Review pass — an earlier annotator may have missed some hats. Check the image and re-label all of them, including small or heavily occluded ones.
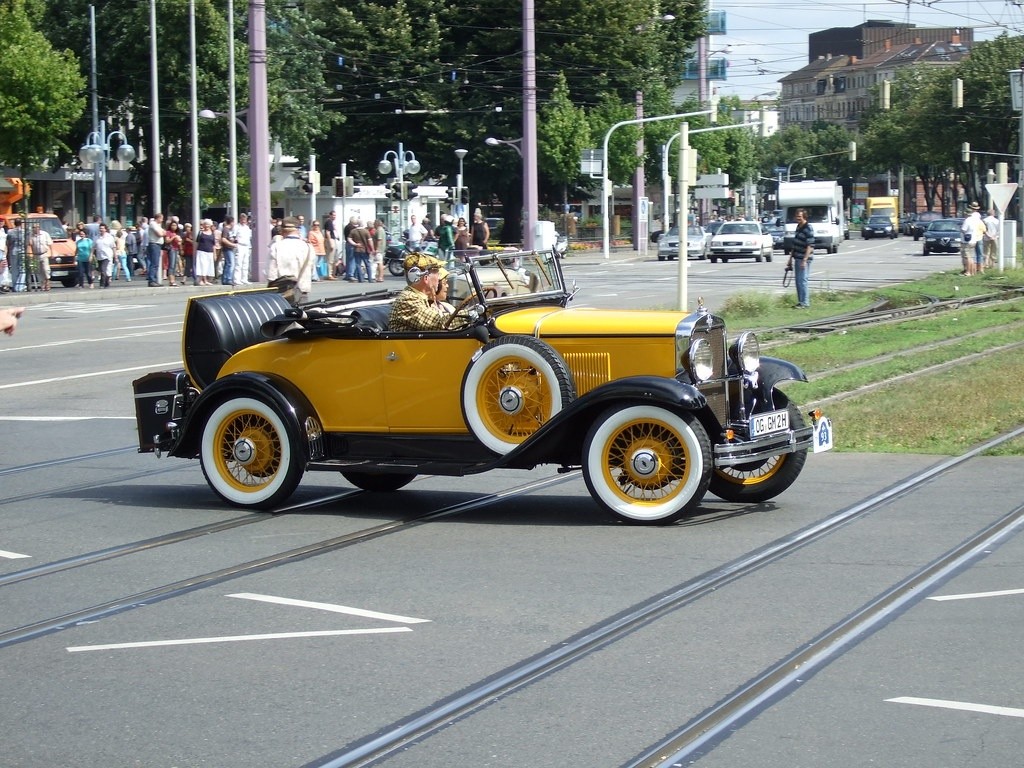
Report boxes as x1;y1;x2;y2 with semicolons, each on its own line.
969;201;981;209
964;208;974;215
444;215;455;223
402;251;448;270
281;216;302;229
110;220;122;229
438;267;450;280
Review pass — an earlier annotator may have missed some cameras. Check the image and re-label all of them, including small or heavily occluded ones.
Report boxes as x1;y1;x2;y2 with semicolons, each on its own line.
785;266;793;272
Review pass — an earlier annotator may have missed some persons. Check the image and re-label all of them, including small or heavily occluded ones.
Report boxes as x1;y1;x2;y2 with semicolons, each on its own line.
905;210;922;222
388;254;485;331
688;196;774;235
656;215;662;222
958;202;999;277
408;207;498;271
267;210;386;283
0;210;254;295
506;257;532;295
787;208;815;310
0;307;26;336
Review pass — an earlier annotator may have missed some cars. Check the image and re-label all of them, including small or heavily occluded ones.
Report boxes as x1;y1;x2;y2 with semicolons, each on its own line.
132;245;833;527
656;210;785;264
844;211;967;255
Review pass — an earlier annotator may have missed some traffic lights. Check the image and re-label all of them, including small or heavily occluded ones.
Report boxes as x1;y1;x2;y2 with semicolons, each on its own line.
403;181;418;201
445;187;455;205
297;171;320;194
392;182;404;200
460;187;469;205
346;177;363;196
385;183;396;201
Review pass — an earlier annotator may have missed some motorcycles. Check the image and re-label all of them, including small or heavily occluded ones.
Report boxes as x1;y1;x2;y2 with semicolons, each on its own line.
383;229;440;276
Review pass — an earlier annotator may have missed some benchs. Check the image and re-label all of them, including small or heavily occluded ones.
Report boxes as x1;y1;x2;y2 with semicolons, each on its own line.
351;303;394;332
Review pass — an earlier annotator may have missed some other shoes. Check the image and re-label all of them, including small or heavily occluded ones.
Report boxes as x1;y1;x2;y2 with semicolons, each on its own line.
0;287;10;293
42;287;51;291
169;282;177;286
976;269;985;275
181;281;185;285
199;281;213;285
139;269;147;274
222;280;236;285
148;281;160;287
959;271;970;276
347;276;358;281
792;302;809;308
233;280;243;284
241;280;253;284
328;274;338;280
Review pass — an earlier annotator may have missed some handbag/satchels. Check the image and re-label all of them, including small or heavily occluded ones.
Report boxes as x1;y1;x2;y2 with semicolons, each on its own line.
964;233;972;241
161;241;172;251
268;274;302;306
365;244;371;254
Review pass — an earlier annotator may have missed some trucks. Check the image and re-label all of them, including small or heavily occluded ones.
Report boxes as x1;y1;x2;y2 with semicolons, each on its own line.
0;177;80;288
863;197;899;238
778;179;845;254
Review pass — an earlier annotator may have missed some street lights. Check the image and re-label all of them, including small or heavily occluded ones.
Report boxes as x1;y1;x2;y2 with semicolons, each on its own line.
485;138;539;251
455;149;468;188
700;47;734;103
79;120;136;225
198;109;271;284
379;143;420;237
